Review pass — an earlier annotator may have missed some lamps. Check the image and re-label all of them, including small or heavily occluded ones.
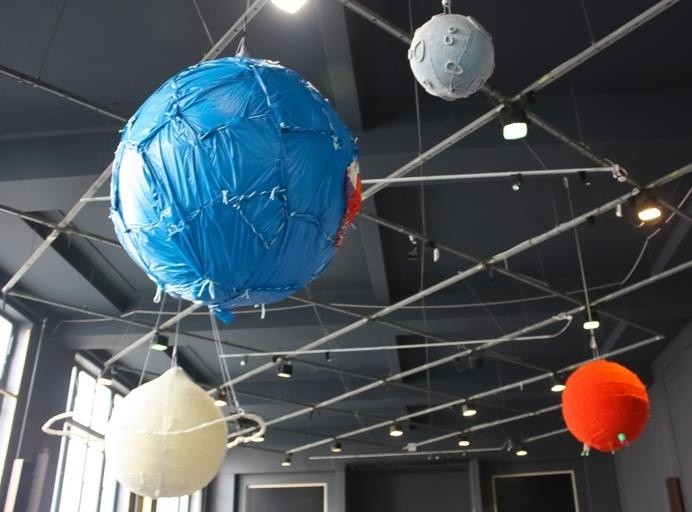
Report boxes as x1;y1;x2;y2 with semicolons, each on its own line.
149;0;662;469
